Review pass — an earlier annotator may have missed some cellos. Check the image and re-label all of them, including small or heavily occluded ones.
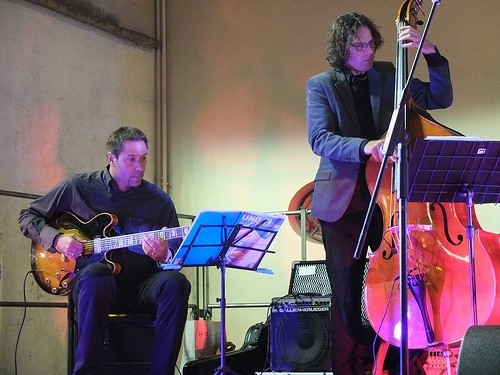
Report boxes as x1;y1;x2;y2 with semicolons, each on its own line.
361;0;500;373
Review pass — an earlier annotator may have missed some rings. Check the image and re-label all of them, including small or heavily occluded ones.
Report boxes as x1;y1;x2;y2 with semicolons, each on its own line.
154;248;159;250
72;253;75;257
151;251;157;255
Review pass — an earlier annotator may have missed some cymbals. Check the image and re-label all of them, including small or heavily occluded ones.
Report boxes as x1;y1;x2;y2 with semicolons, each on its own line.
287;180;324;245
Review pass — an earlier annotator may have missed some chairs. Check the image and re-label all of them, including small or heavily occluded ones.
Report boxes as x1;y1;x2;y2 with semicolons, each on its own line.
68;295;158;375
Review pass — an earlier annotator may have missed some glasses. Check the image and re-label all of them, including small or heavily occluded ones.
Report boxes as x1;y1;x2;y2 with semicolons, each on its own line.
349;38;377;52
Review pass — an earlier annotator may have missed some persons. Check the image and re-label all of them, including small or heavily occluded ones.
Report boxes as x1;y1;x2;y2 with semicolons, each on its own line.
305;12;453;375
17;125;192;375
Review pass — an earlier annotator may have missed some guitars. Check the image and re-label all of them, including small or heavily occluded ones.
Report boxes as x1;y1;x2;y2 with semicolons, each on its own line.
29;208;193;297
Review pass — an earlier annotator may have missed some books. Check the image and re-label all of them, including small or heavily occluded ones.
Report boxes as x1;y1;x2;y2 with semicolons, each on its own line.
169;211;285;268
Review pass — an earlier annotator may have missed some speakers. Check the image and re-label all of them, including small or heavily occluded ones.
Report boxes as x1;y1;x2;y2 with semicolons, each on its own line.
456;324;500;375
270;258;380;372
